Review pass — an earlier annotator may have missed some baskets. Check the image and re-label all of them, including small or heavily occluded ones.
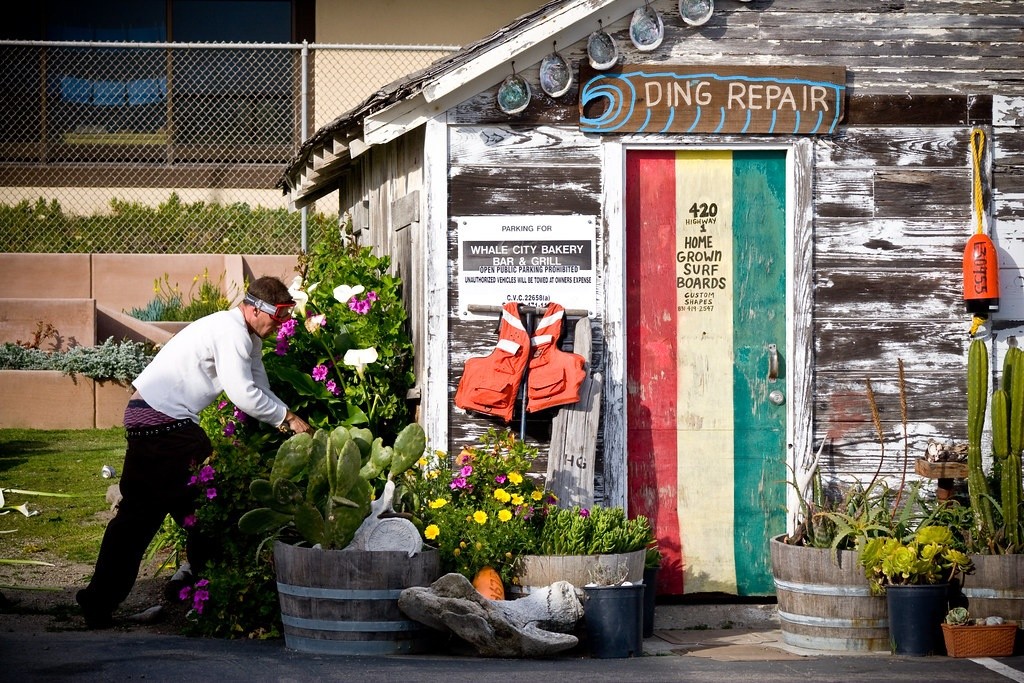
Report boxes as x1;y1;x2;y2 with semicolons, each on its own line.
941;618;1019;657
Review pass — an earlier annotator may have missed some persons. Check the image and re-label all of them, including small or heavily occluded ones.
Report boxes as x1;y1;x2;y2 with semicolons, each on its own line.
76;276;315;623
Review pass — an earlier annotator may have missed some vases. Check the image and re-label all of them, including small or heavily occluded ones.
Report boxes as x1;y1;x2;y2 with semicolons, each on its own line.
472;566;505;600
583;585;647;659
145;321;193;336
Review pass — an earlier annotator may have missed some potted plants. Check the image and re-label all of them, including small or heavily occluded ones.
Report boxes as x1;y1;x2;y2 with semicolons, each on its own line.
954;340;1024;629
0;320;165;429
860;525;974;657
513;505;663;600
770;448;925;652
941;607;1018;657
237;422;441;657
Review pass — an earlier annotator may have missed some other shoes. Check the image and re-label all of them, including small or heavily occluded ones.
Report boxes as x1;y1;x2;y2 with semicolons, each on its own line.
76;589;113;628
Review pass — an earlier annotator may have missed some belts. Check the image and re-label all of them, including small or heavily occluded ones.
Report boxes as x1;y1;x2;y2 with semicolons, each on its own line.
128;419;192;438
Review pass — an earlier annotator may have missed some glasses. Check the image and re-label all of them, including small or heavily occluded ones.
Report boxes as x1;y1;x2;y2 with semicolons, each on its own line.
245;291;296;323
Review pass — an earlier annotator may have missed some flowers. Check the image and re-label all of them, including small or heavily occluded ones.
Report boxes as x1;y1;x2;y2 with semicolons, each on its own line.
410;426;560;585
153;270;251;321
